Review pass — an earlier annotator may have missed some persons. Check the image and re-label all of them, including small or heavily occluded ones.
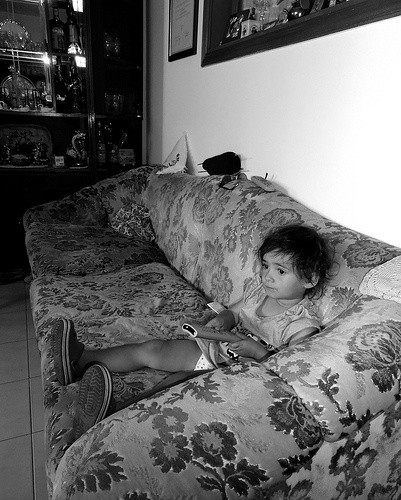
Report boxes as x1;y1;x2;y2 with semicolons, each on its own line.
231;20;240;33
52;226;331;442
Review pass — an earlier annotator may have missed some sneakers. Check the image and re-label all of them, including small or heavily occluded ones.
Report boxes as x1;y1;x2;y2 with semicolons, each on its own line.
72;363;114;440
51;318;85;386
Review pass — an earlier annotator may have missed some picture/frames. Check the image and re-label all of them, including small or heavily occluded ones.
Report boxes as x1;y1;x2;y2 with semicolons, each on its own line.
167;0;199;62
200;0;401;67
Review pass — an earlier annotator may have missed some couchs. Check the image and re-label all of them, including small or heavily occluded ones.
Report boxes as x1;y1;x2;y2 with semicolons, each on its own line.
24;163;401;500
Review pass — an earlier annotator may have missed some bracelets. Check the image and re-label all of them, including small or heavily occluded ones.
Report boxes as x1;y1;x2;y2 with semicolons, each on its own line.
257;352;271;363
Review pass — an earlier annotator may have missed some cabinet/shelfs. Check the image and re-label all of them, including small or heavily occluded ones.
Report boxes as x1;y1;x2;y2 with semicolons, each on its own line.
0;0;150;283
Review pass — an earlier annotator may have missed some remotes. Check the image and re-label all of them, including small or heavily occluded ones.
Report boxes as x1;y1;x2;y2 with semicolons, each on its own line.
251;175;277;192
181;322;241;342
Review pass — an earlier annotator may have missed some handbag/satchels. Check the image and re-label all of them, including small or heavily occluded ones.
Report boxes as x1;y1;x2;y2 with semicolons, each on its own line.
202;152;247;190
